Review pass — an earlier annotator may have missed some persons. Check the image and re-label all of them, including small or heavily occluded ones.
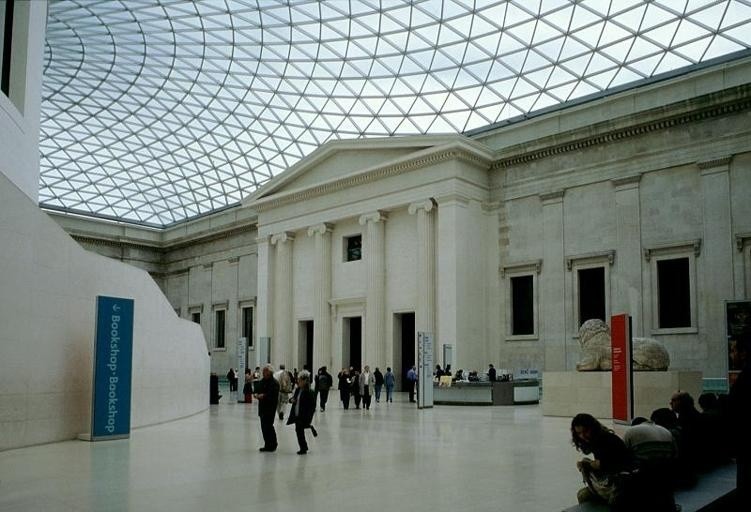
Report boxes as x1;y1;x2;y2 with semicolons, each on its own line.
570;335;751;512
227;363;496;455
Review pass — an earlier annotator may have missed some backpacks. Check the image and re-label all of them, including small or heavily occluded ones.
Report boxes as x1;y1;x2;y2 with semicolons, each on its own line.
279;371;294;393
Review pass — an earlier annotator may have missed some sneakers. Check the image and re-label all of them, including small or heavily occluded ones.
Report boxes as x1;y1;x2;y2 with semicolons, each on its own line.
279;411;284;420
321;397;417;412
297;446;309;455
260;442;278;453
